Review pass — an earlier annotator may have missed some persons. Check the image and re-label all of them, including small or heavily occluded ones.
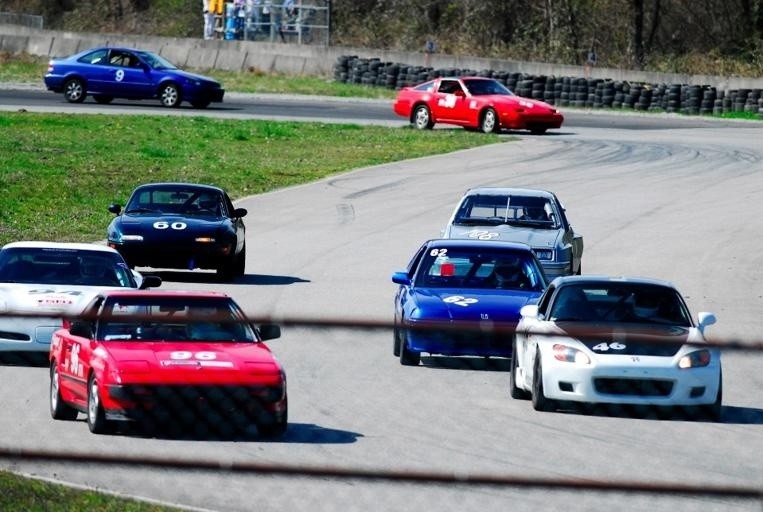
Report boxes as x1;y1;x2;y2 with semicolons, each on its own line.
493;254;527;290
182;305;219;335
202;0;318;46
631;292;662;320
520;200;545;223
67;253;102;278
195;193;220;213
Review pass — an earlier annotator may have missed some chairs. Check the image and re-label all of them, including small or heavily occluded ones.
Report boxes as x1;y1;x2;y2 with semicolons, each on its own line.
520;211;548;220
564;293;591;319
67;270;120;286
484;273;532;288
8;261;32;279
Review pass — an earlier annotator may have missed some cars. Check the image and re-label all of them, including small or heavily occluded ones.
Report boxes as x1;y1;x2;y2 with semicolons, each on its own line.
50;290;287;438
509;276;722;415
106;182;246;279
1;241;160;352
392;239;549;365
442;187;582;284
393;77;563;134
44;46;225;108
